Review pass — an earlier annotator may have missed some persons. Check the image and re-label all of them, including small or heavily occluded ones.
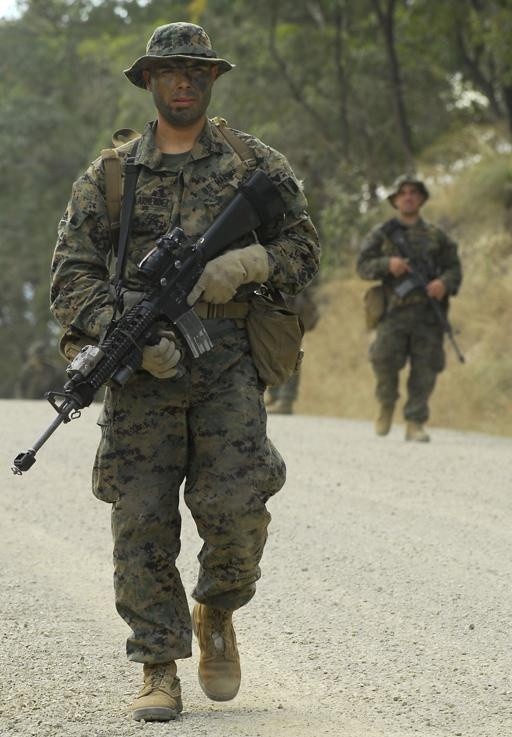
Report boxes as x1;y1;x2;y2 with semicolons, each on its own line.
264;291;306;414
48;21;324;723
355;173;463;441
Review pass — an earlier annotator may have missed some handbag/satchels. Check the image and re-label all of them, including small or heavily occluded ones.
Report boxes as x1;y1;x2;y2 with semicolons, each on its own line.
363;284;387;325
247;291;307;389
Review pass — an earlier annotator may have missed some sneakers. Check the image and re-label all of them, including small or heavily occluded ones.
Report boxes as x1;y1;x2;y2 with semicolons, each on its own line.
265;396;298;415
191;601;241;702
374;403;396;436
405;419;429;441
132;660;185;723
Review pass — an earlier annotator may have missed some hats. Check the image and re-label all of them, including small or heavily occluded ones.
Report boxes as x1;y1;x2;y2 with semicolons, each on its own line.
385;174;431;211
121;20;236;90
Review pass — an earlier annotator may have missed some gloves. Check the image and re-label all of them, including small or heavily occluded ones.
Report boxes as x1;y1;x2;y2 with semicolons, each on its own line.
140;336;185;380
186;241;271;309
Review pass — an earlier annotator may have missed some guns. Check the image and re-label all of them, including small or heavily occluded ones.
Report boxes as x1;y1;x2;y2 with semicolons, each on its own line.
13;171;286;477
381;216;467;364
